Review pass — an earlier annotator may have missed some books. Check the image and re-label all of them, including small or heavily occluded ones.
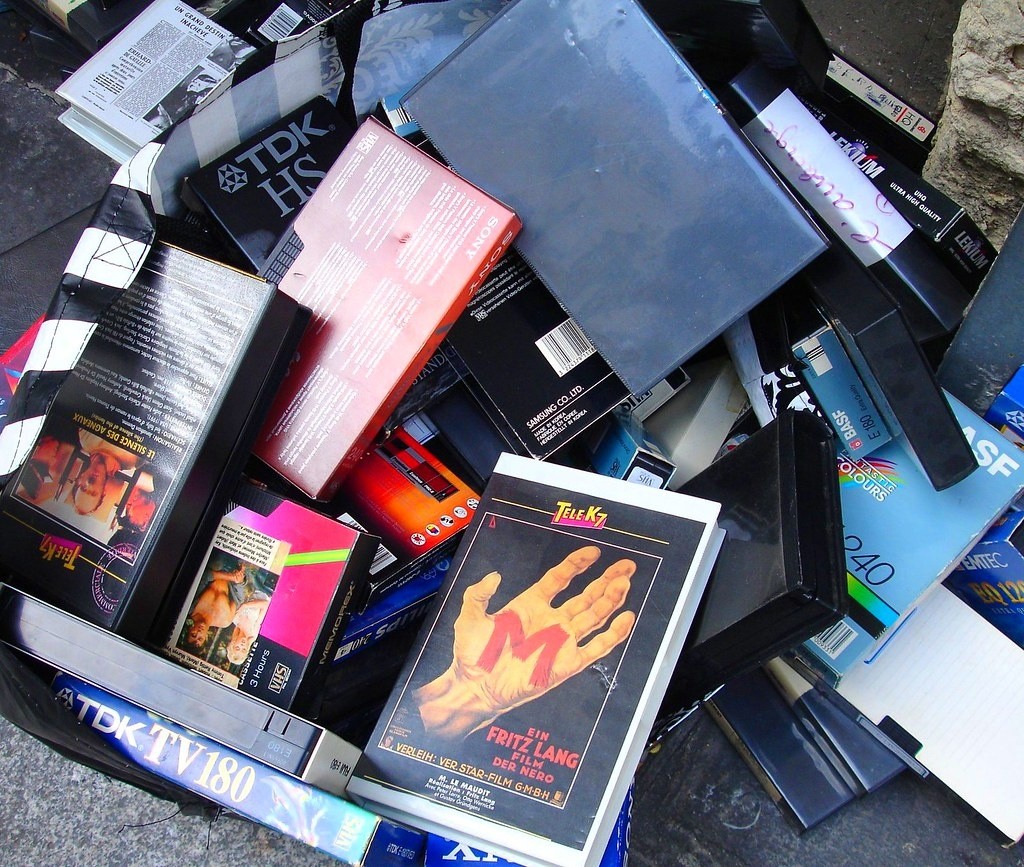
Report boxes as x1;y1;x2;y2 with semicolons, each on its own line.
1;0;1024;867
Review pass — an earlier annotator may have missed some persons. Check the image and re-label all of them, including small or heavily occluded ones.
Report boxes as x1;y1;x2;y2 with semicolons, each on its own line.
32;413;172;530
415;546;637;744
187;550;278;666
